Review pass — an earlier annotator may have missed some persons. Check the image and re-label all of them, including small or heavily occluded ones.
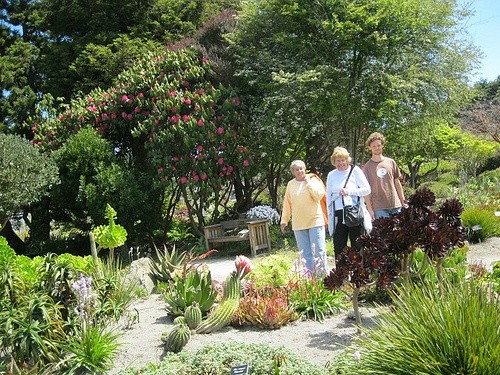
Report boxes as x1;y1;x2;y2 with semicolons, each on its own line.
280;160;327;281
326;146;371;270
361;131;409;221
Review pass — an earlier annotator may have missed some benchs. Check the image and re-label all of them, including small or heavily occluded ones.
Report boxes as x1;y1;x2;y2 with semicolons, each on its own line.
203;218;272;256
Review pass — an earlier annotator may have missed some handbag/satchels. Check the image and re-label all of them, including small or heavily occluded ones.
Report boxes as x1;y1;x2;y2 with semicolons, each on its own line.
344;203;364;228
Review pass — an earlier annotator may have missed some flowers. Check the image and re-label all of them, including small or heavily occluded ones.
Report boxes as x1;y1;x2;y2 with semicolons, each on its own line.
247;205;280;224
